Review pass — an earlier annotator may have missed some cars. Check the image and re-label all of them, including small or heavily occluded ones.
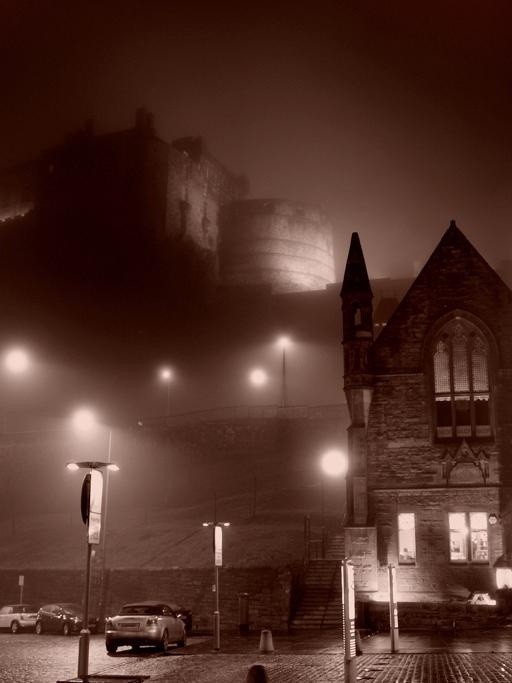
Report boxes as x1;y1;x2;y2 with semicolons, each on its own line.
104;601;187;653
0;603;40;633
166;602;192;637
35;602;100;636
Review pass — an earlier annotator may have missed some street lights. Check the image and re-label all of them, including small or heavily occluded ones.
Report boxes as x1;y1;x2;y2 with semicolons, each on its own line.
65;461;120;680
163;369;171;416
280;336;290;406
72;408;112;633
321;448;344;559
202;520;231;650
